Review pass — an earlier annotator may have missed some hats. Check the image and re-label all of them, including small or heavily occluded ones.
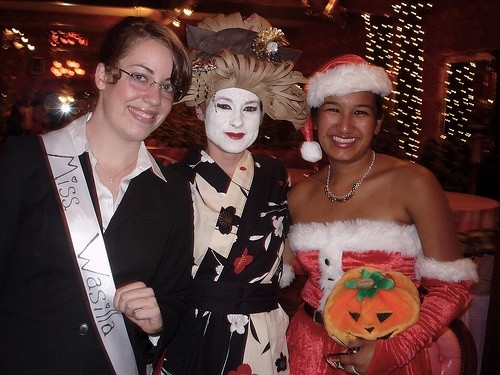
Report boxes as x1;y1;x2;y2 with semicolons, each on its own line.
300;56;393;163
172;13;310;131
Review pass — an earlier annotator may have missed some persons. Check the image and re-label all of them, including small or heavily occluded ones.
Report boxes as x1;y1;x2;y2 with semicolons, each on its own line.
279;54;479;375
0;16;194;375
173;48;311;375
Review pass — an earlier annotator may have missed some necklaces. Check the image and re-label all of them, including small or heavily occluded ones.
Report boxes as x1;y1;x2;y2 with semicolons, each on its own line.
94;155;138;185
324;150;376;203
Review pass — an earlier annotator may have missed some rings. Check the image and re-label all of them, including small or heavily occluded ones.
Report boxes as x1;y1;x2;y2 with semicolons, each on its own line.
352;365;360;375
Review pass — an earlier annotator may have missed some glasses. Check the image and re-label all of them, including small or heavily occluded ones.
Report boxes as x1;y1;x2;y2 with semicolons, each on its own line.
115;66;186;102
